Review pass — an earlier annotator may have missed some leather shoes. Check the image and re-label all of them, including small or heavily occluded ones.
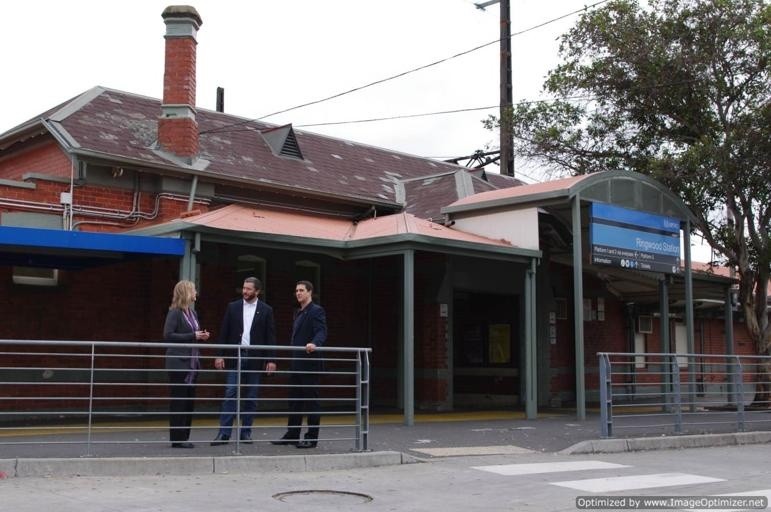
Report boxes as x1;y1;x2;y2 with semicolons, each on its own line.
270;436;299;446
296;436;318;448
240;432;253;444
210;432;229;446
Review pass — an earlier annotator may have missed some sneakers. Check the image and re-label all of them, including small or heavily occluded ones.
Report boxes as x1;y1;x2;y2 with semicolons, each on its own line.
172;439;195;448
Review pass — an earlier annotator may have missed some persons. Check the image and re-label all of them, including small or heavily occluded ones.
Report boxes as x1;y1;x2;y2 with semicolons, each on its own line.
163;280;211;448
271;280;329;448
210;277;278;445
